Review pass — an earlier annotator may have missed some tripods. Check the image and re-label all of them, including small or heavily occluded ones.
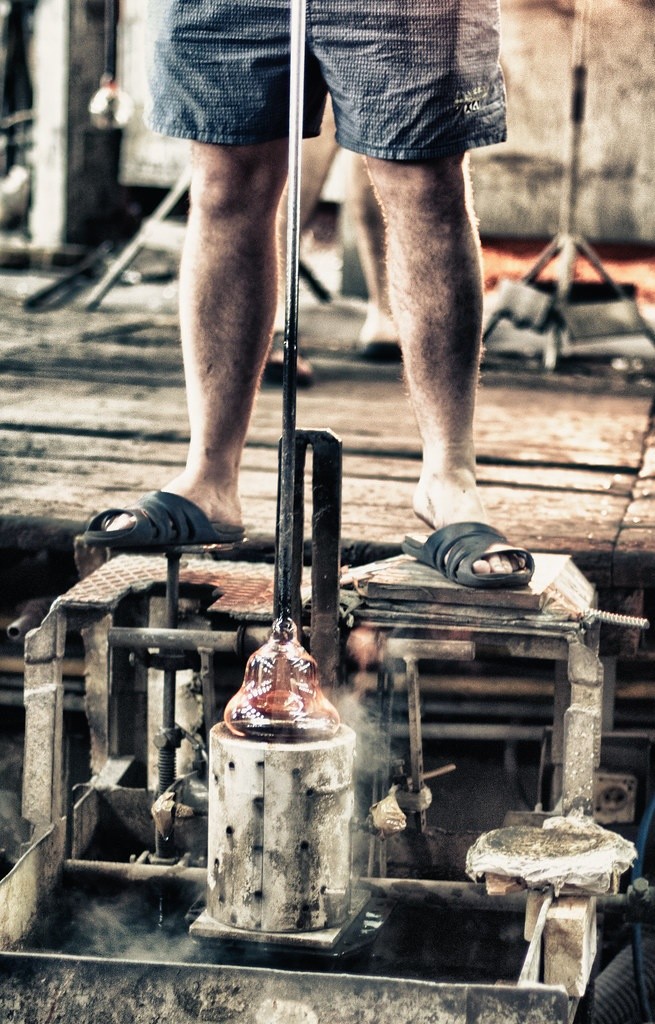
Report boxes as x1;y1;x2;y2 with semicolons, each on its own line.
478;0;655;372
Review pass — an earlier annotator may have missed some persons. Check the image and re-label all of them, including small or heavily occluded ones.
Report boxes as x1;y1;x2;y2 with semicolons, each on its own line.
75;0;536;588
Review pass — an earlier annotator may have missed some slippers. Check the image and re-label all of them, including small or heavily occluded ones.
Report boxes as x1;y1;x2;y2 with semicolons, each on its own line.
402;521;535;587
84;490;246;548
